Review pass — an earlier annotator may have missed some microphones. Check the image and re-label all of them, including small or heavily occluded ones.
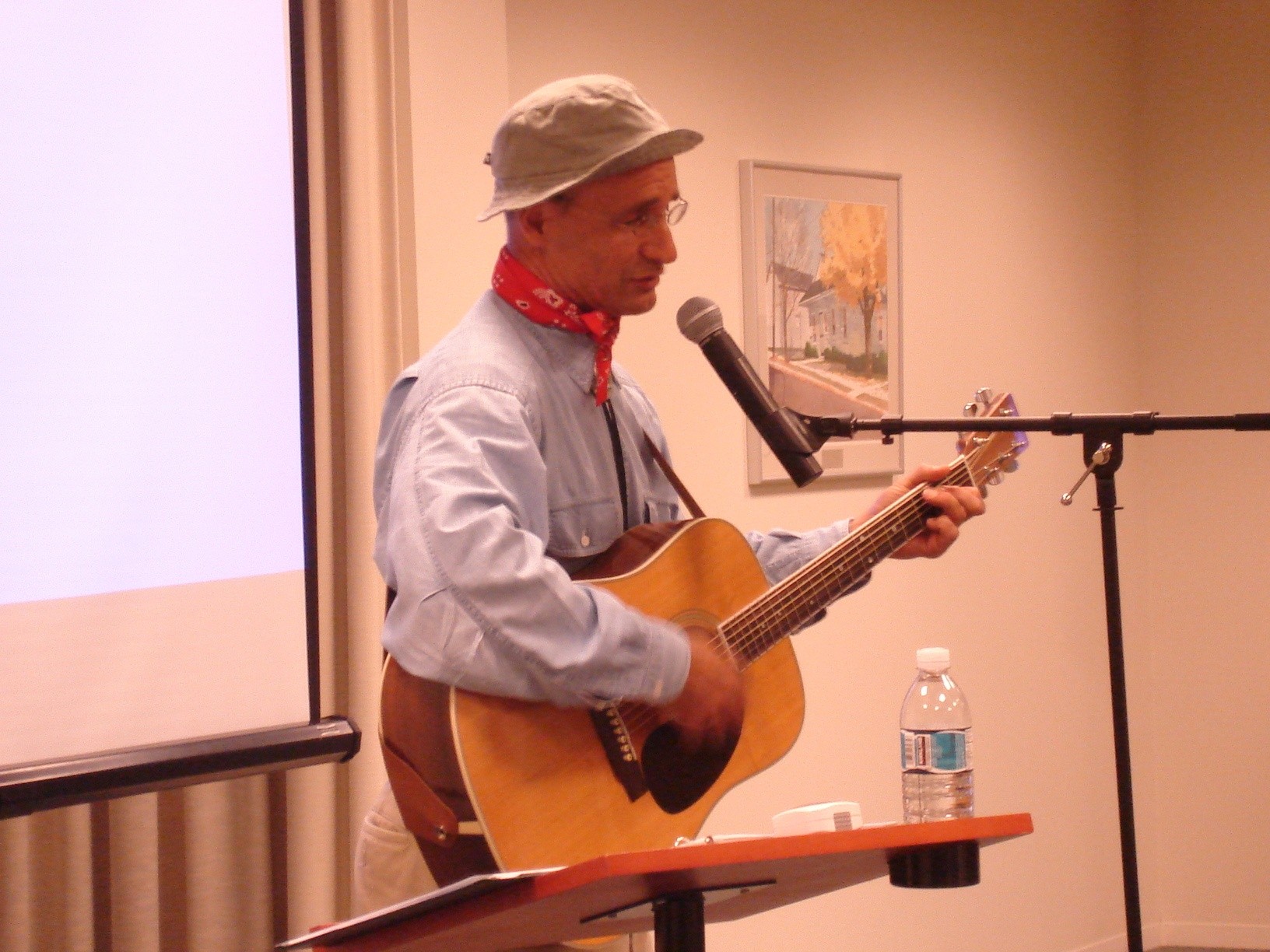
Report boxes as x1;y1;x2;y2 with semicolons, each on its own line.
677;297;823;488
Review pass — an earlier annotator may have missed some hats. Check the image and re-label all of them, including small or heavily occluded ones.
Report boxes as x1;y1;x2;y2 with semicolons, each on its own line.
478;75;704;223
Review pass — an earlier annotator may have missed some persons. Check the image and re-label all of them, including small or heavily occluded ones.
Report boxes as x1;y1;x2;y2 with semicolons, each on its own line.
375;72;987;889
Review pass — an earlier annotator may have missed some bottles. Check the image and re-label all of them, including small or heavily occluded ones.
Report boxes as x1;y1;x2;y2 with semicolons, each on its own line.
899;649;977;822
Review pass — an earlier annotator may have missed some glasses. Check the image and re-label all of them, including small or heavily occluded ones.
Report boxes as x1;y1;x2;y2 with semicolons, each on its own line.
557;194;689;236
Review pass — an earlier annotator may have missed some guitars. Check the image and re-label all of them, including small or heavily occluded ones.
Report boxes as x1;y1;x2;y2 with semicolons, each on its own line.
378;389;1032;888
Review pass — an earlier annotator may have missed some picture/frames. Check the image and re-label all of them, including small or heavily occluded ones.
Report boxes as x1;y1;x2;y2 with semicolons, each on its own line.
737;158;906;486
